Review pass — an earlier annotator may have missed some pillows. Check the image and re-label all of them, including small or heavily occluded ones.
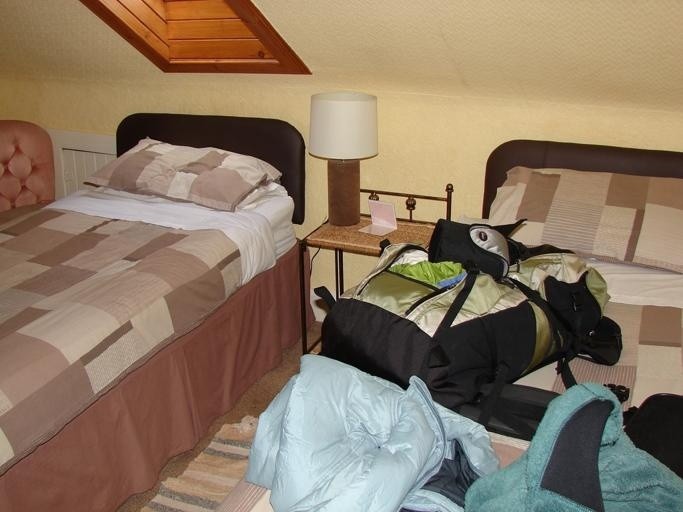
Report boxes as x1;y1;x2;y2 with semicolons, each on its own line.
83;136;282;212
489;166;682;275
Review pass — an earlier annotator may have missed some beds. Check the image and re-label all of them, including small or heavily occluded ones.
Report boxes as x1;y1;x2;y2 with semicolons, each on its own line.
218;139;683;512
1;112;316;512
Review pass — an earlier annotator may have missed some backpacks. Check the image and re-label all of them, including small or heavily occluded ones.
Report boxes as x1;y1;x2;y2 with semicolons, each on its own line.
312;217;629;409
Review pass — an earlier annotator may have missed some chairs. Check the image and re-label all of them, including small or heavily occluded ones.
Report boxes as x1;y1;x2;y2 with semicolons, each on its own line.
2;119;55;214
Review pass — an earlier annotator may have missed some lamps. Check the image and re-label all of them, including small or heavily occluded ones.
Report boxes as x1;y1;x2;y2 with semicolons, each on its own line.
308;90;379;227
